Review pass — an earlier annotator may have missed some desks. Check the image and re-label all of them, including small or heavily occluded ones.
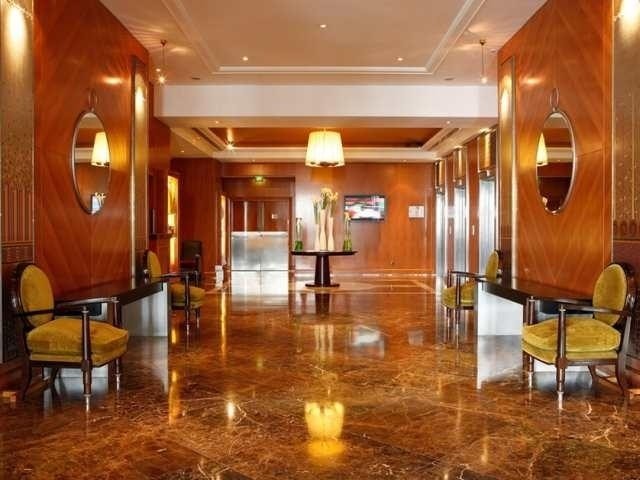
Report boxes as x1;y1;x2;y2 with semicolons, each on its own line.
52;275;165;378
473;275;595;371
288;284;356;329
291;250;360;289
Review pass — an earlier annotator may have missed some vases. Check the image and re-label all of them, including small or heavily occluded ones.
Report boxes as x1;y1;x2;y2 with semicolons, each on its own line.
327;216;335;250
318;209;328;250
314;223;320;251
295;224;303;251
343;224;352;251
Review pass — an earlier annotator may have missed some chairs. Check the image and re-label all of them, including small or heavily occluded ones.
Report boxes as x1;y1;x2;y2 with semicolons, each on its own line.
521;260;638;397
142;248;205;329
11;261;130;399
179;237;203;272
438;248;505;321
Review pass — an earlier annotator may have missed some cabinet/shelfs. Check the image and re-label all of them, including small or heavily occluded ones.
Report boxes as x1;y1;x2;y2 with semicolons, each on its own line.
232;199;289;232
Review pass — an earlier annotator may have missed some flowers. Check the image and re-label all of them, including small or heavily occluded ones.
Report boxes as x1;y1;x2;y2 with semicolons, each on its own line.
319;187;331;208
330;191;339;217
310;194;321;224
344;212;352;222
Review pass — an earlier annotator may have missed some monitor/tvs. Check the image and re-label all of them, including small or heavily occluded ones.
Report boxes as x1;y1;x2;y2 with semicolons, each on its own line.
343;194;386;220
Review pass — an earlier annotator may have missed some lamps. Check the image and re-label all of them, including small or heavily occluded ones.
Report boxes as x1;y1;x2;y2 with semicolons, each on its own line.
302;398;348;441
536;130;549;167
304;127;348;170
90;130;110;168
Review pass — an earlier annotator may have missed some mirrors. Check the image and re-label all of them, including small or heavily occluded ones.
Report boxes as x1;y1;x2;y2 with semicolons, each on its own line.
70;110;115;215
128;51;152;280
534;108;580;217
